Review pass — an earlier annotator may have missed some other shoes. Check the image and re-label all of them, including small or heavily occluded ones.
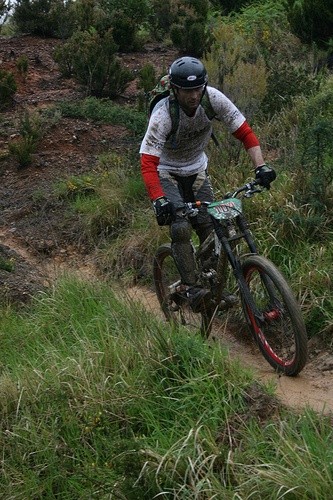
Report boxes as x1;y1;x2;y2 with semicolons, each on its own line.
217;295;238;311
186;286;210;313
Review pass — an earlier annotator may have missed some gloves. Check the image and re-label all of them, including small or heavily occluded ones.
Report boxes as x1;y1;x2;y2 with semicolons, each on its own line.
153;196;176;226
254;164;276;190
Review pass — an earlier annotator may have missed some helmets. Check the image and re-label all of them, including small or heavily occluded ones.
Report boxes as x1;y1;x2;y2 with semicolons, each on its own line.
168;56;207;89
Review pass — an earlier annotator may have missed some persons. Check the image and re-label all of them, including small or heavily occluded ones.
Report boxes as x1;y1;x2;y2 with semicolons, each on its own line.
139;56;276;314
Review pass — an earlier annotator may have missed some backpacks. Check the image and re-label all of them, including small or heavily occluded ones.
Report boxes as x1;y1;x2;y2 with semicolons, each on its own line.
143;74;216;140
